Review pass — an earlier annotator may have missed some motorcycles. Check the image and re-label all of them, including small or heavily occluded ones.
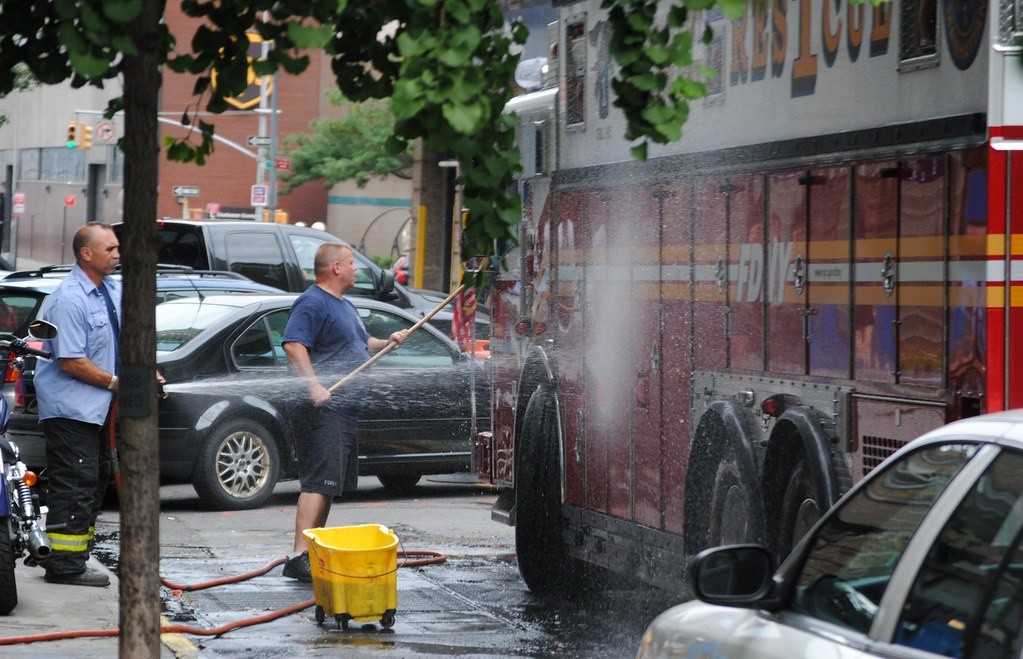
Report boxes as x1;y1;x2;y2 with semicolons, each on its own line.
0;320;58;618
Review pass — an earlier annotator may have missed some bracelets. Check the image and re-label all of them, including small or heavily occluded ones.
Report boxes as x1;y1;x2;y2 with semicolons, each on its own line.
108;376;118;390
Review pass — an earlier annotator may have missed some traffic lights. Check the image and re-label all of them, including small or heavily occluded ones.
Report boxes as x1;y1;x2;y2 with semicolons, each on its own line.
81;124;92;150
66;123;76;150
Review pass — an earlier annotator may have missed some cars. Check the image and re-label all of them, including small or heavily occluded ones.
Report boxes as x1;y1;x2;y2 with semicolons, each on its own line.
6;293;492;510
635;406;1022;658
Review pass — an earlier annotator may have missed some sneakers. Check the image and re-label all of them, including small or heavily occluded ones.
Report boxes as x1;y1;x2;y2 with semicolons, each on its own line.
283;552;315;582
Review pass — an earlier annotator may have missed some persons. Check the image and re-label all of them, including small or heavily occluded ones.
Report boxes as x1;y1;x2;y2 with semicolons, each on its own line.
33;222;169;586
279;243;409;583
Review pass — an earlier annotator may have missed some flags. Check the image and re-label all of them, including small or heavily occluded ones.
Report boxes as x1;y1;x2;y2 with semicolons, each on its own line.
450;260;478;352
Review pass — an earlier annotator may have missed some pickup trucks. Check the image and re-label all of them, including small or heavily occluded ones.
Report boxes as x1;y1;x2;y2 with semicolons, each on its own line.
109;218;491;358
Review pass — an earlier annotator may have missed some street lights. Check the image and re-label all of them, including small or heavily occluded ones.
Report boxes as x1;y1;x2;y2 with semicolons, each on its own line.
438;160;463;262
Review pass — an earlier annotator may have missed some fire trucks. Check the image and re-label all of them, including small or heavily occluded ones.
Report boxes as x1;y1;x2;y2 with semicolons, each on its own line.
478;1;1023;611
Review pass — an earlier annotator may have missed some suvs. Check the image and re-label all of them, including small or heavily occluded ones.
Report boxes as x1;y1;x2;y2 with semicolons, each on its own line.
0;263;291;390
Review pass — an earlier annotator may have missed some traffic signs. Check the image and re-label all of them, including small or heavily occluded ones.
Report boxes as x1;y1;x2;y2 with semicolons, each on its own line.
246;135;271;146
172;186;198;196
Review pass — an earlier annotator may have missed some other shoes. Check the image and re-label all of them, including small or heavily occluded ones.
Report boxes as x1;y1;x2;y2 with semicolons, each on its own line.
44;566;109;587
